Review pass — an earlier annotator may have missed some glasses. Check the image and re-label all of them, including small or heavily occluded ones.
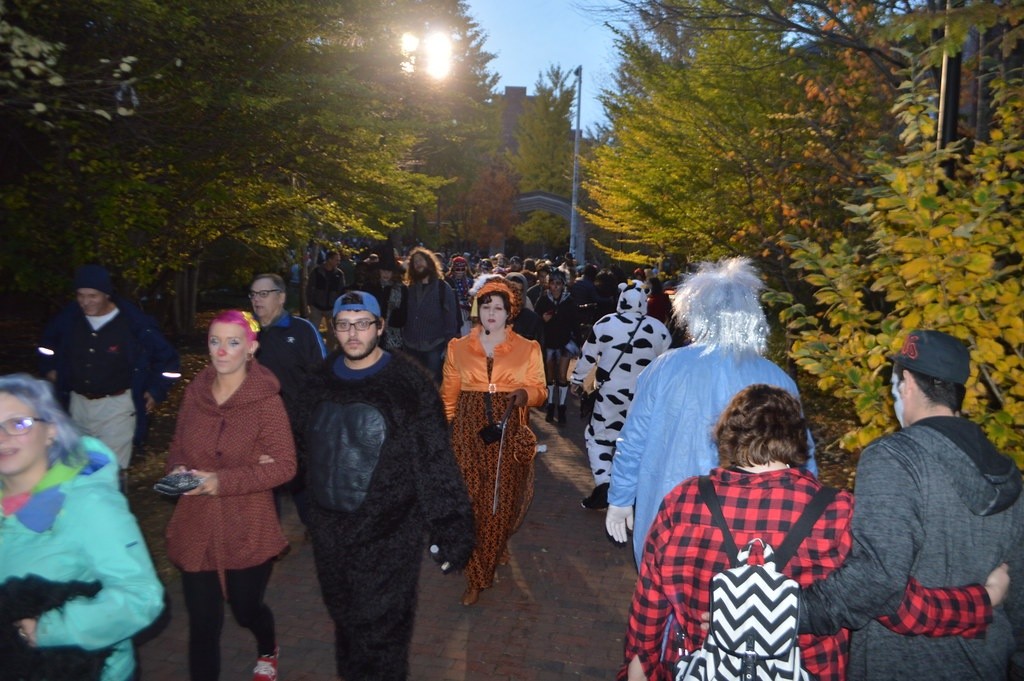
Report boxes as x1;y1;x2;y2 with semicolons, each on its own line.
455;268;465;271
334;321;376;332
249;289;280;299
0;416;46;435
551;281;562;285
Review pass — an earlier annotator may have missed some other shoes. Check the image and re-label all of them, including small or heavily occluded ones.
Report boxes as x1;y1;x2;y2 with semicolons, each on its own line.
462;588;478;605
499;543;509;565
583;482;610;509
581;387;600;417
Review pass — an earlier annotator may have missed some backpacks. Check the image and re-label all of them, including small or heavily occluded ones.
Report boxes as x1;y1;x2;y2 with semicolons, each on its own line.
675;475;840;681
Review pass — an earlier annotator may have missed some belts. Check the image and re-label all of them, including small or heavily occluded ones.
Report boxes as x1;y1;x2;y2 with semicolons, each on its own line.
74;386;130;400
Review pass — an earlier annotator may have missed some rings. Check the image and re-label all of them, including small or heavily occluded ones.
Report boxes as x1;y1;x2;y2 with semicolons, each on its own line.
19;628;26;639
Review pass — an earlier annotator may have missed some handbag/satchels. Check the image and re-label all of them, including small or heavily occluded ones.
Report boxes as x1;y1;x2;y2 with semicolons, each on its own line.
514;406;537;465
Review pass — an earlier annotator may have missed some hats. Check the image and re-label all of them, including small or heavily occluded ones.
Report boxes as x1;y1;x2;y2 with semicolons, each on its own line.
333;291;380;317
510;256;522;264
369;247;406;273
469;276;525;322
72;264;114;295
890;331;969;384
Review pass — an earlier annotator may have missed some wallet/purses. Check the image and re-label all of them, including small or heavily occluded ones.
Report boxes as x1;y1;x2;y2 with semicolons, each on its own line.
154;472;202;495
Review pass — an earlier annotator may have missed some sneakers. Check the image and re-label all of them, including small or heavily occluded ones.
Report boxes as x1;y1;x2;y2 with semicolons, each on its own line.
253;641;280;681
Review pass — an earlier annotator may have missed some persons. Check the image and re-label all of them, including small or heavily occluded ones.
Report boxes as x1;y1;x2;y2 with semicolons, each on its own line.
40;266;180;497
605;254;818;662
249;272;326;527
796;329;1024;681
281;234;673;428
624;383;1010;681
570;280;672;511
162;311;296;681
288;289;475;681
0;374;166;681
439;273;550;605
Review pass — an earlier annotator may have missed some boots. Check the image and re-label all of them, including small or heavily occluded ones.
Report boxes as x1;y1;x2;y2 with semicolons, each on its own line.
545;403;555;423
557;404;567;424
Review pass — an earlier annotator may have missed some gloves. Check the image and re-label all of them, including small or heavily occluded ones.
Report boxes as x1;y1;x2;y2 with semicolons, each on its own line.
508;389;527;408
